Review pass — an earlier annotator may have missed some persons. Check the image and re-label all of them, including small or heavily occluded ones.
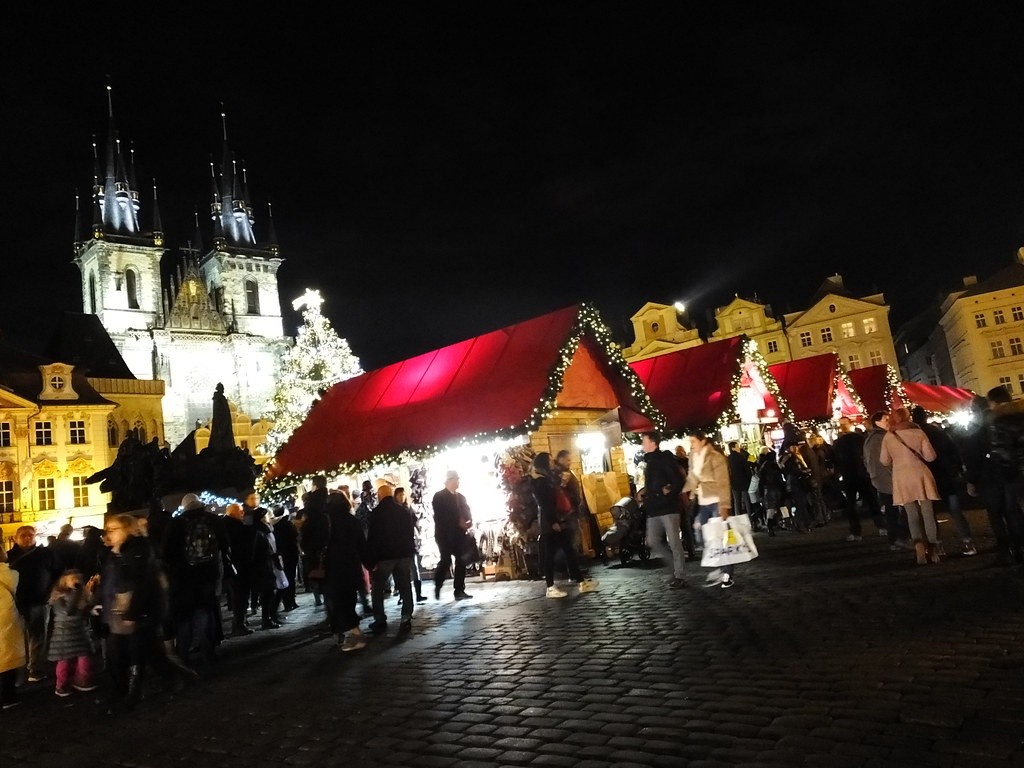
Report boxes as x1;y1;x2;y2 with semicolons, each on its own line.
753;444;785;535
301;474;378;651
555;450;589;582
112;430;143;468
833;417;887;541
394;487;428;605
367;485;416;635
432;469;473;600
142;436;159;466
727;442;762;531
529;453;601;597
0;493;299;697
854;408;941;564
676;445;702;562
777;434;846;533
208;383;236;449
688;429;734;588
912;386;1024;561
641;430;688;588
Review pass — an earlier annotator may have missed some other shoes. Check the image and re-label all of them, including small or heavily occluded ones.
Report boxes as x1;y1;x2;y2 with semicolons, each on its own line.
764;517;1024;564
2;698;22;709
70;679;98;691
434;584;441;599
454;588;472;599
28;670;48;682
400;620;413;630
54;687;74;697
370;621;389;633
232;596;373;649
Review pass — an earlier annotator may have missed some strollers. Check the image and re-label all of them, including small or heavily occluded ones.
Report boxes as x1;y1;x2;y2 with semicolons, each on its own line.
600;486;650;567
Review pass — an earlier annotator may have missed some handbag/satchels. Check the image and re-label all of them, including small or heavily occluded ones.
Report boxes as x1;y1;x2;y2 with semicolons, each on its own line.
457;529;480;565
700;512;758;567
320;539;339;571
927;458;951;498
271;558;289;589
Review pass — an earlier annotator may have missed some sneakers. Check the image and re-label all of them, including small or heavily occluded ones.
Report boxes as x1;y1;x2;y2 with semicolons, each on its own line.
721;573;735;588
545;585;568;597
578;579;600;593
702;568;725;587
669;578;688;589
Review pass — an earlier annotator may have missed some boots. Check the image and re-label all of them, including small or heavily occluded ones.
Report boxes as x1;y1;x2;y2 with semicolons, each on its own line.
414;579;428;602
157;657;199;696
397;597;404;605
120;665;143;712
